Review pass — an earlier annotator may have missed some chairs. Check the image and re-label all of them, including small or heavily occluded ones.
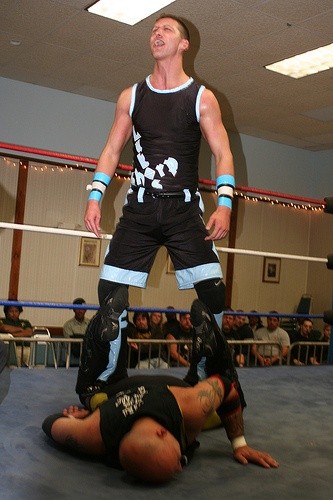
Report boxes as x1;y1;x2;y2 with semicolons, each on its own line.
32;328;57;369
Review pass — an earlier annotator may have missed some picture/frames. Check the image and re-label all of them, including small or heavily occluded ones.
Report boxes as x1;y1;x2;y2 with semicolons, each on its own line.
166;251;176;273
262;256;281;283
79;236;102;267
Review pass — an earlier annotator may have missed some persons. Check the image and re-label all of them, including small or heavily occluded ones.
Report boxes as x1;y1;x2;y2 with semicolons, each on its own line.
63;296;333;366
42;373;280;480
0;296;33;365
74;15;248;415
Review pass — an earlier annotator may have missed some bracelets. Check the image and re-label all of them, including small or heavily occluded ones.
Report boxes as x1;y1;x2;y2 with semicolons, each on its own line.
231;435;246;450
